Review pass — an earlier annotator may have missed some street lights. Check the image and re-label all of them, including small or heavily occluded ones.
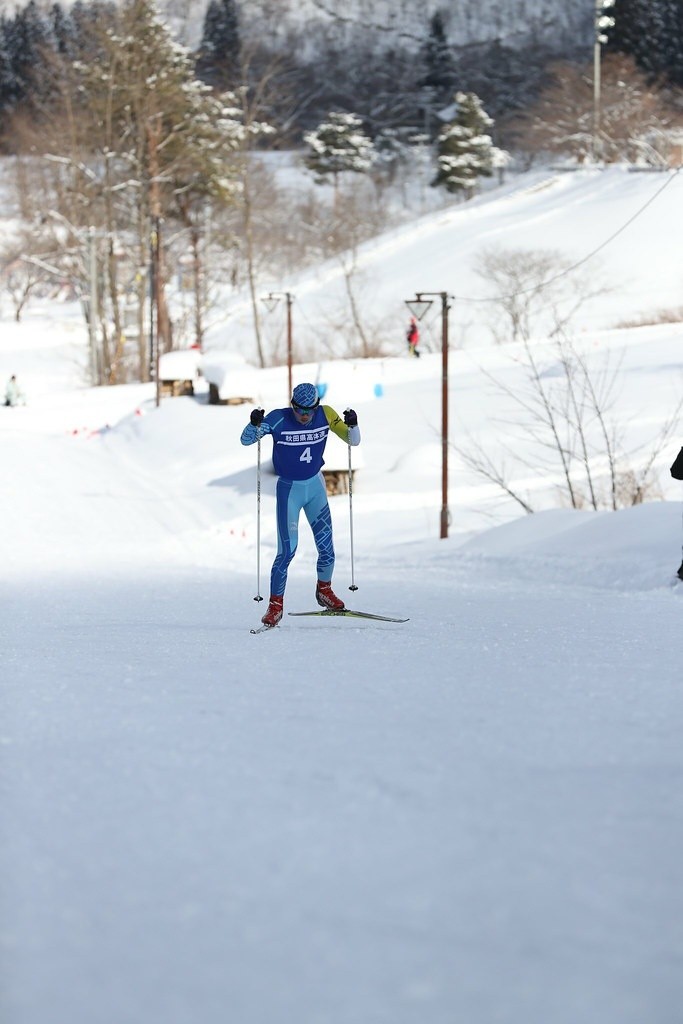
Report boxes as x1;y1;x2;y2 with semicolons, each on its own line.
404;292;450;539
259;291;294;408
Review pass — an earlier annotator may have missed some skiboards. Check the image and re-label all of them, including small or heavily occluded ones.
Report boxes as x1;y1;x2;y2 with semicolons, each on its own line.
249;610;410;635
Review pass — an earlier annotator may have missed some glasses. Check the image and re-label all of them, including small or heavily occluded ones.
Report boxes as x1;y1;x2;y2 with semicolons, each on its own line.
291;398;320;416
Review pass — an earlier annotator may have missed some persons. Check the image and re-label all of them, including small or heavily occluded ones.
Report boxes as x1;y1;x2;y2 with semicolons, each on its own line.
669;445;683;582
240;382;362;626
4;375;24;408
405;315;421;359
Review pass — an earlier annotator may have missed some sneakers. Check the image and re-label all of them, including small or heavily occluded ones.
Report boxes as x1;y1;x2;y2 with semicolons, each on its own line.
262;595;283;626
316;580;345;610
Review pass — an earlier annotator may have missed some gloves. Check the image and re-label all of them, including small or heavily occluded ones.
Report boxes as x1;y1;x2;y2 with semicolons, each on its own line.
343;409;358;429
250;409;265;426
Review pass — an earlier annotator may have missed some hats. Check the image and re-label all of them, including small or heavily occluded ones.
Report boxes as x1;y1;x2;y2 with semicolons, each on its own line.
291;383;319;410
